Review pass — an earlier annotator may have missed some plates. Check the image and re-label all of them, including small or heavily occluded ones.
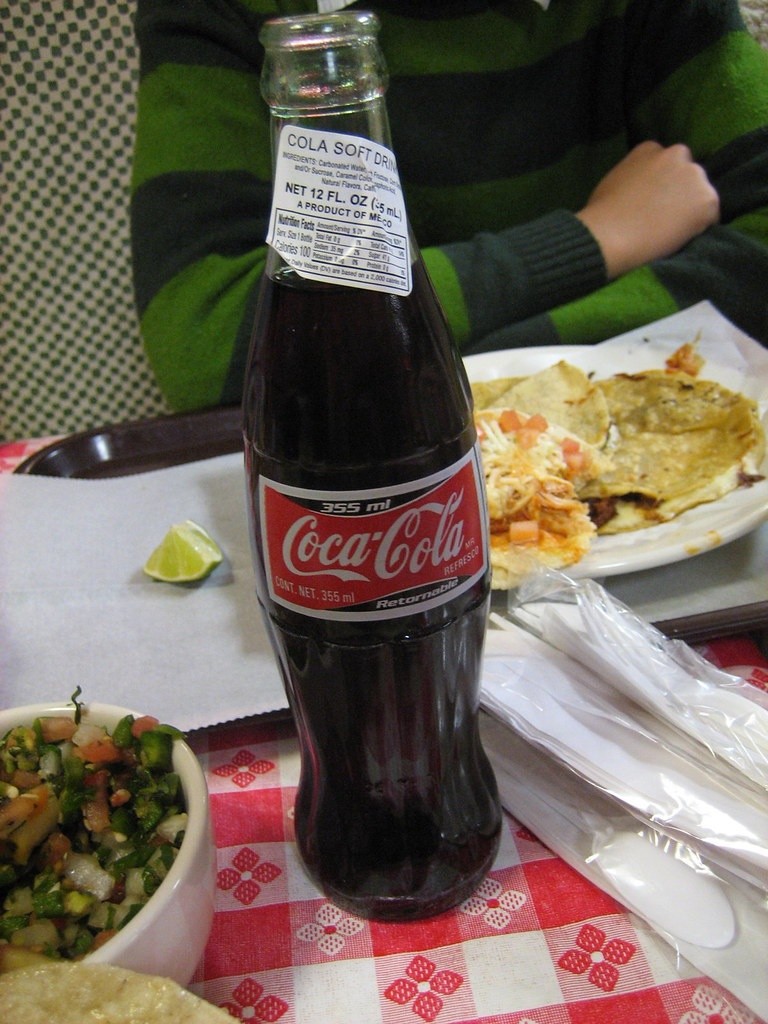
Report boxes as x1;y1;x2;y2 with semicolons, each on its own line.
462;344;768;581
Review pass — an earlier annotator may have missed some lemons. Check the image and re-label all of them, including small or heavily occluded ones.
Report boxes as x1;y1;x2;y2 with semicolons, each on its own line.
143;518;222;582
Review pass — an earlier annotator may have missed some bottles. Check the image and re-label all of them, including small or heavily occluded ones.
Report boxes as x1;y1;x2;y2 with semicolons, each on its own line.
244;9;501;920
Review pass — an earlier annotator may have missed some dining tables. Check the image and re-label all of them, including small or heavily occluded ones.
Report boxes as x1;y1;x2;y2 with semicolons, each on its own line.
0;433;768;1024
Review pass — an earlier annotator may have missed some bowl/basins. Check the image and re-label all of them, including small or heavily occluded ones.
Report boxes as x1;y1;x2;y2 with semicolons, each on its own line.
0;700;216;988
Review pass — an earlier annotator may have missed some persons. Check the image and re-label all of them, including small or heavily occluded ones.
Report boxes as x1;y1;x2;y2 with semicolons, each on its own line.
127;0;768;417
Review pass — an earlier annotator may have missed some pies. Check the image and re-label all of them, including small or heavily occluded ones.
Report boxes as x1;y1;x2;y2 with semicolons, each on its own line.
473;342;766;593
